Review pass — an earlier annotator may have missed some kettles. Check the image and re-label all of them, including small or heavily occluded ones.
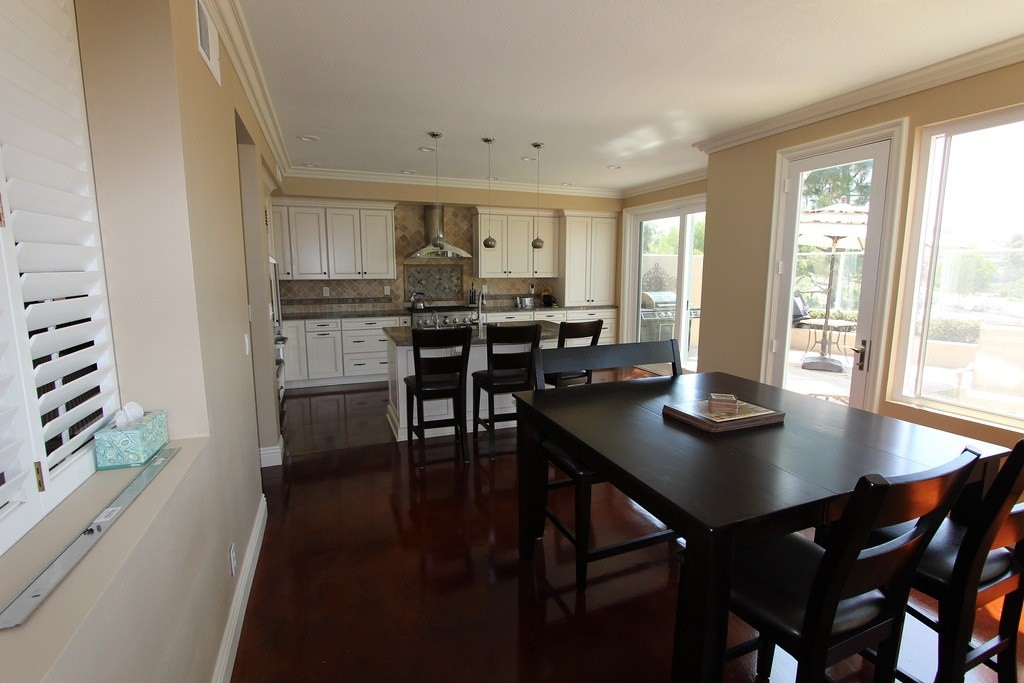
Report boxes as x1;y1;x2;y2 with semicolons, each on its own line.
409;291;425;309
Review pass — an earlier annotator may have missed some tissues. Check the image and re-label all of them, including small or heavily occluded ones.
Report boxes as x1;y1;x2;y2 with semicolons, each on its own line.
93;401;170;471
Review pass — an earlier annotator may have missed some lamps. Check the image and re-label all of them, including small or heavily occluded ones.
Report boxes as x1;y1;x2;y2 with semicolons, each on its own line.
532;143;544;249
429;132;444;247
481;137;497;248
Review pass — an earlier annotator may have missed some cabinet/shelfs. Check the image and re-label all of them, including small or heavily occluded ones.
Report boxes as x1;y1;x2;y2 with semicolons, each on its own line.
271;196;399;279
469;205;620;308
382;320;562;444
282;306;618;381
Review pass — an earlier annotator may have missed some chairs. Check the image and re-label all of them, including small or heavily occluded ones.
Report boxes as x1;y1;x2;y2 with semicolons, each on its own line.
403;318;1024;683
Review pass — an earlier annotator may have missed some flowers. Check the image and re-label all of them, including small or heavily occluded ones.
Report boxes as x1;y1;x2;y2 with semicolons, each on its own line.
541;283;558;304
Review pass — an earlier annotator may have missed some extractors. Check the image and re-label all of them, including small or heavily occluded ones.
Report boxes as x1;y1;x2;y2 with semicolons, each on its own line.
405;204;472;258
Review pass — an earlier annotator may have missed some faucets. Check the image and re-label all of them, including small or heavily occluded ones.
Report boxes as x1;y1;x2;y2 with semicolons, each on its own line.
431;310;438;330
471;291;486;328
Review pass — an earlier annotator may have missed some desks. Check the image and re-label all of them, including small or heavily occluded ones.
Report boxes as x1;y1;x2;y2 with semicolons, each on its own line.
512;371;1014;683
800;319;857;364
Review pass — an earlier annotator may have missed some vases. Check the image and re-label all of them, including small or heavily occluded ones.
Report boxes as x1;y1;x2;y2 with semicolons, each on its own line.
543;300;552;308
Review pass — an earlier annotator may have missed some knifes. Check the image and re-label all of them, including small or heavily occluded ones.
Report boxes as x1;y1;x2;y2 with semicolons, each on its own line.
469;283;476;304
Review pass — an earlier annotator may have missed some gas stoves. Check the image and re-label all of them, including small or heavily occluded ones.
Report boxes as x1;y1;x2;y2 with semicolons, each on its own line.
407;305;478;327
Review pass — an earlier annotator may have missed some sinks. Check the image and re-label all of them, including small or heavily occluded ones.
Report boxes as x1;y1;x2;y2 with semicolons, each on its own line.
422;327;454;330
460;325;487;329
